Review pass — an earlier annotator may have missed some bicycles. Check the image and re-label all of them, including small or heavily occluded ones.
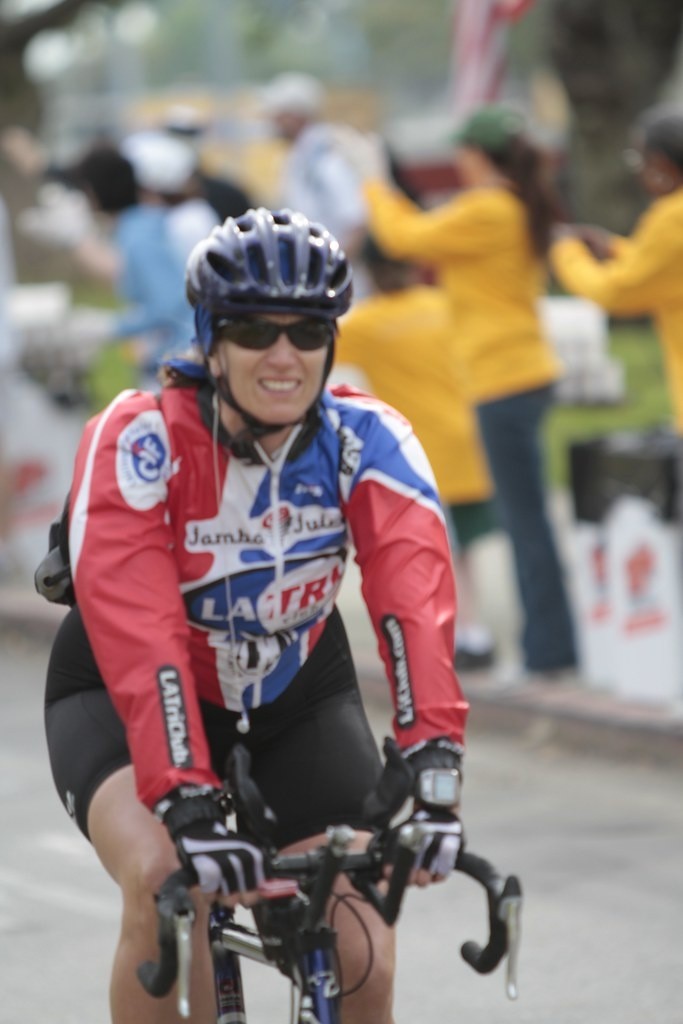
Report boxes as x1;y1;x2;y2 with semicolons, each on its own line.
135;824;523;1024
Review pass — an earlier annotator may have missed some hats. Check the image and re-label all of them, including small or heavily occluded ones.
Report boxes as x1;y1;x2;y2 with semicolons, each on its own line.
255;70;326;116
117;131;202;195
449;108;519;151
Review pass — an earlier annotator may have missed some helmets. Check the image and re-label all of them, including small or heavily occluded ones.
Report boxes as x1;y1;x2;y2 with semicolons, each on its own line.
182;206;355;360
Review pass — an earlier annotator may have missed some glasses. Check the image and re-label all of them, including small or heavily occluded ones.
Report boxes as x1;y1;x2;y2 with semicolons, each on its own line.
216;313;330;352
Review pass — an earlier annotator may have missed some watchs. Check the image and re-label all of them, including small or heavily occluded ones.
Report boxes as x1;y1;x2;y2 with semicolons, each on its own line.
413;765;465;809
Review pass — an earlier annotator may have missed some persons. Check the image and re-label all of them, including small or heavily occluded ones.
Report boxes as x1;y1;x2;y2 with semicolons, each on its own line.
0;75;581;695
546;114;683;521
45;208;470;1024
